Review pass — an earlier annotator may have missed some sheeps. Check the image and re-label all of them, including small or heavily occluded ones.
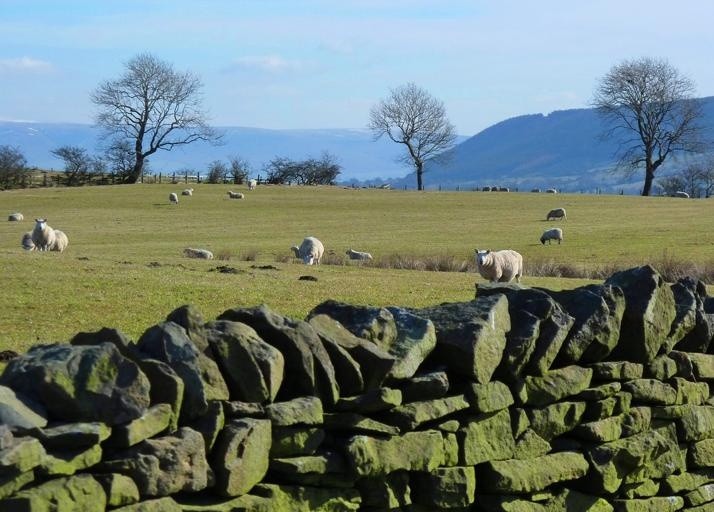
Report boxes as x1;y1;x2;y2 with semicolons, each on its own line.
183;246;215;260
169;178;257;204
291;233;324;267
483;186;509;192
473;247;524;285
7;212;70;253
546;208;567;221
675;191;690;198
531;188;558;194
540;228;563;246
346;248;373;261
